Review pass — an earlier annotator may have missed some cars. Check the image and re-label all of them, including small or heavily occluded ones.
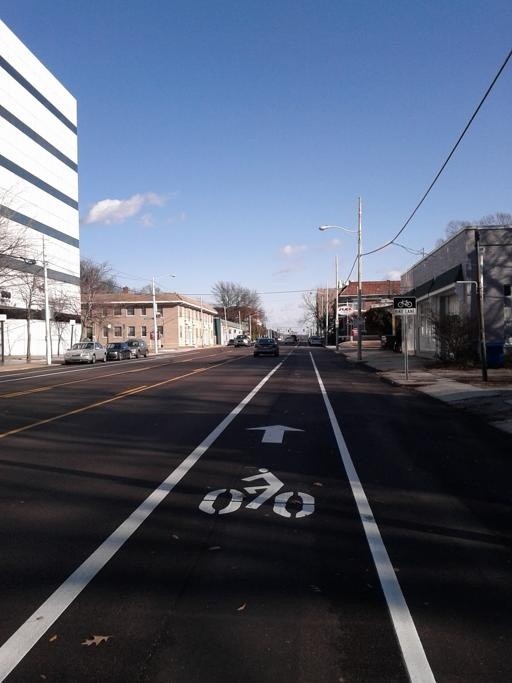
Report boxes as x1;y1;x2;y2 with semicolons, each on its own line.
253;338;279;357
64;338;150;364
285;335;299;345
308;336;323;346
228;334;256;347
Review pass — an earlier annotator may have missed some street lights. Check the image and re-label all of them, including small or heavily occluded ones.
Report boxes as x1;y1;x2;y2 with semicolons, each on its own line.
152;274;176;353
319;197;362;361
310;280;330;345
